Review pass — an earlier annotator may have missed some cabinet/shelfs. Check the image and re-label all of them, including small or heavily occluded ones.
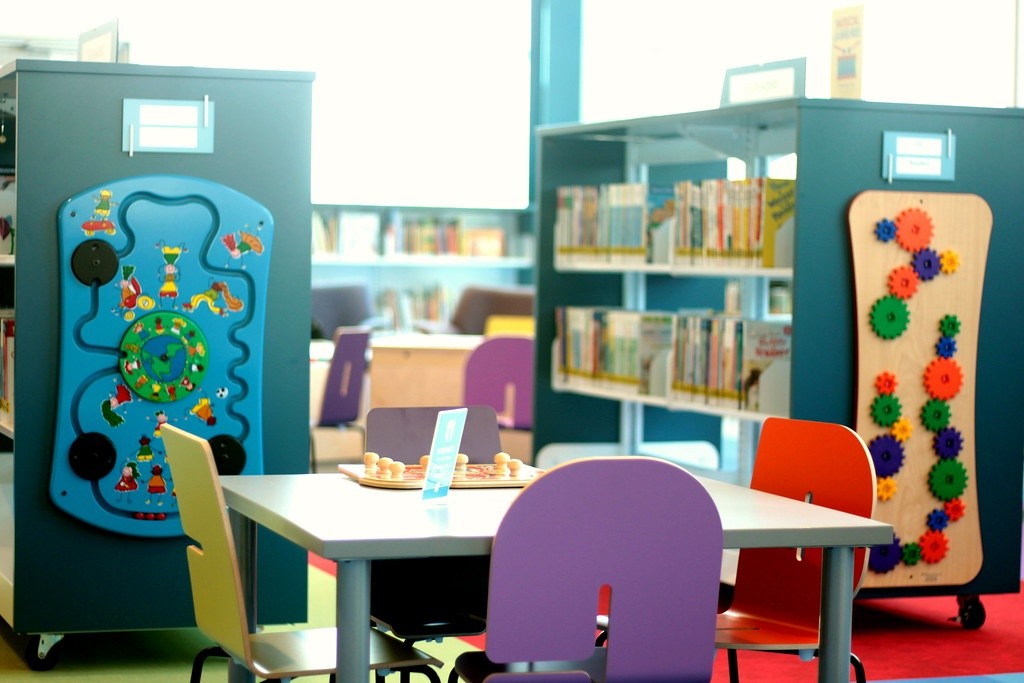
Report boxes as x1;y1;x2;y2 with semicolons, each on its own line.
0;58;316;673
533;97;1023;624
308;204;536;360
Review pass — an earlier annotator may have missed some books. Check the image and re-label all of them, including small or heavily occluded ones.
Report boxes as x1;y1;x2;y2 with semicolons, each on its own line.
555;178;795;267
0;308;15;427
311;210;503;331
554;305;791;411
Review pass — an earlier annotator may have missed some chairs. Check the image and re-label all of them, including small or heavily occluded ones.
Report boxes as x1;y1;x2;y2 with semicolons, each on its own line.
365;398;507;683
302;284;535;474
158;423;445;683
449;455;724;683
593;416;877;683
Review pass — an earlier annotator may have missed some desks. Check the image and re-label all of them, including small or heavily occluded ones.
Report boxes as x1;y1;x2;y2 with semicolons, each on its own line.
309;333;532;463
212;470;895;683
